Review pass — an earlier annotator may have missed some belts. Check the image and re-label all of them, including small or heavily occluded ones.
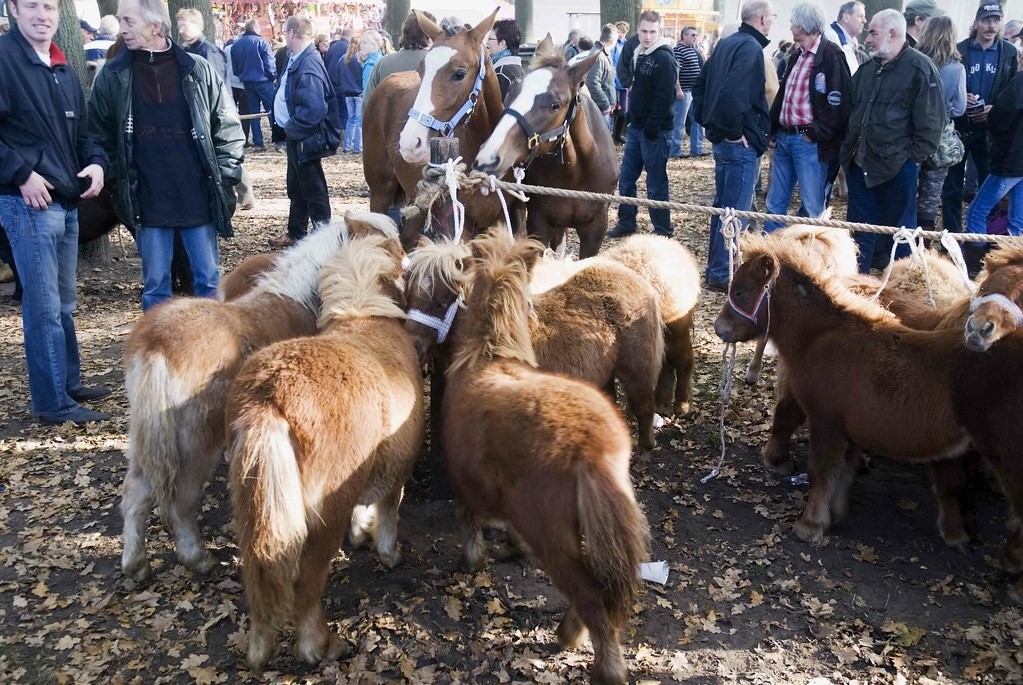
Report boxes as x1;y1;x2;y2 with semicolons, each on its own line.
778;123;811;136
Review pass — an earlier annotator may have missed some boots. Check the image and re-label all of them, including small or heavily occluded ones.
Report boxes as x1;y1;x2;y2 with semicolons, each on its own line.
962;242;986;272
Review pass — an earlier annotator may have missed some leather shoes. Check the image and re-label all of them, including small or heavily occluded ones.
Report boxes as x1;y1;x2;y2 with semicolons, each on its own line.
69;386;111;401
39;405;111;426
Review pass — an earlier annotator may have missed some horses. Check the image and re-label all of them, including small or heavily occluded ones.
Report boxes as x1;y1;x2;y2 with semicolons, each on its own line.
114;6;1023;685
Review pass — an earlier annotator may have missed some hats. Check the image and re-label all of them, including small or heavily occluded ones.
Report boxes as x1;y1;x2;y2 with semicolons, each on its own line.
779;40;792;50
1012;28;1023;38
976;1;1003;20
906;0;946;17
80;19;98;32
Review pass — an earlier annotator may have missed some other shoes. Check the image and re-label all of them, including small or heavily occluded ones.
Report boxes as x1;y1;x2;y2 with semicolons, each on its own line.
709;281;731;293
268;236;296;247
699;152;711;156
679;153;690;158
756;191;767;197
607;227;636;236
244;141;254;148
362;191;371;197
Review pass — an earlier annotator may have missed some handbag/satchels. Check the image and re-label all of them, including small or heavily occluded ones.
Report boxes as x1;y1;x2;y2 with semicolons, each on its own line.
925;119;966;170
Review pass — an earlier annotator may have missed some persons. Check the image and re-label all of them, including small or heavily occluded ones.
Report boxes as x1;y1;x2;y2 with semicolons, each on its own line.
0;0;1023;427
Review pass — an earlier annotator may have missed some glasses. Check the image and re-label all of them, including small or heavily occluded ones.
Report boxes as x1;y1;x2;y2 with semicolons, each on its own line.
282;29;296;35
760;14;778;20
487;36;499;41
692;34;699;37
